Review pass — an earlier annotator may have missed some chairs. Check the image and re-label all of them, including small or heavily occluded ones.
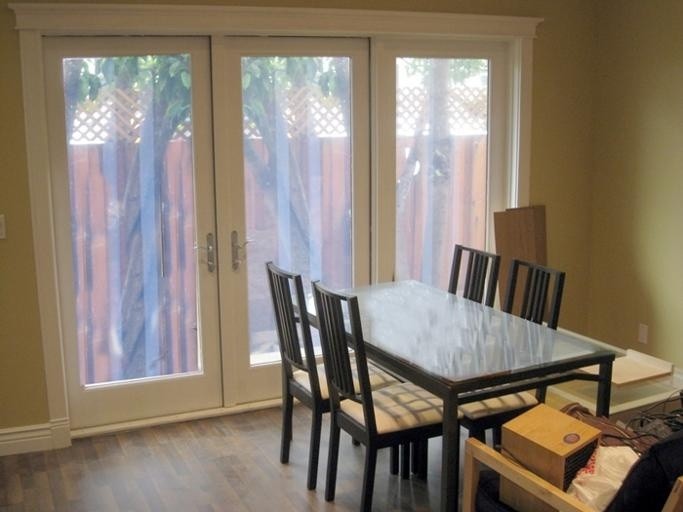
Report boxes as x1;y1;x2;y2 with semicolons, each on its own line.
310;279;464;512
264;259;400;490
447;244;501;309
462;408;683;512
418;257;567;485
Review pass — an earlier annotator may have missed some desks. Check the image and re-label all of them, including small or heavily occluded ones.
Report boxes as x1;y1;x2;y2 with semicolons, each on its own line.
291;278;615;512
